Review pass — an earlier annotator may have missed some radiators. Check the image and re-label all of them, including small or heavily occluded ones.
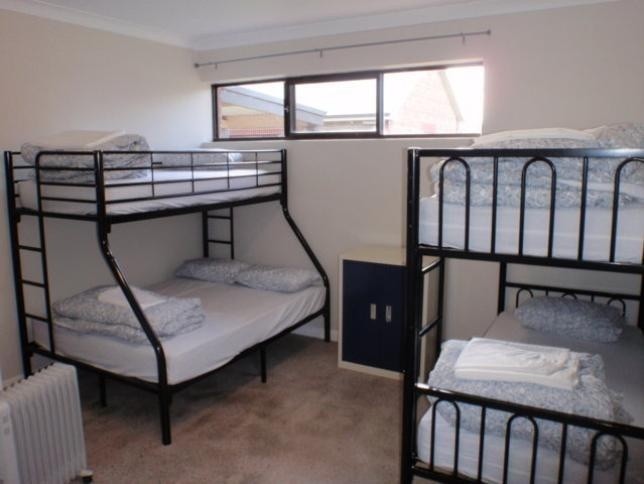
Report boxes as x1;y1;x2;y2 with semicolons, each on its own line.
0;361;88;484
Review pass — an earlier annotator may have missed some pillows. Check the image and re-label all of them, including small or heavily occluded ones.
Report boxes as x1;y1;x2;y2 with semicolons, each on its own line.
172;257;322;293
516;293;625;344
155;148;244;169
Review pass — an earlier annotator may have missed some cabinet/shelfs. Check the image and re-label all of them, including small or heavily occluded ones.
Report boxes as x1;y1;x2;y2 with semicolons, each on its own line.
339;246;440;384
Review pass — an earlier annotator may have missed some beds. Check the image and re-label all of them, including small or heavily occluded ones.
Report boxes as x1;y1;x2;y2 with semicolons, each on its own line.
394;141;642;483
3;147;331;442
